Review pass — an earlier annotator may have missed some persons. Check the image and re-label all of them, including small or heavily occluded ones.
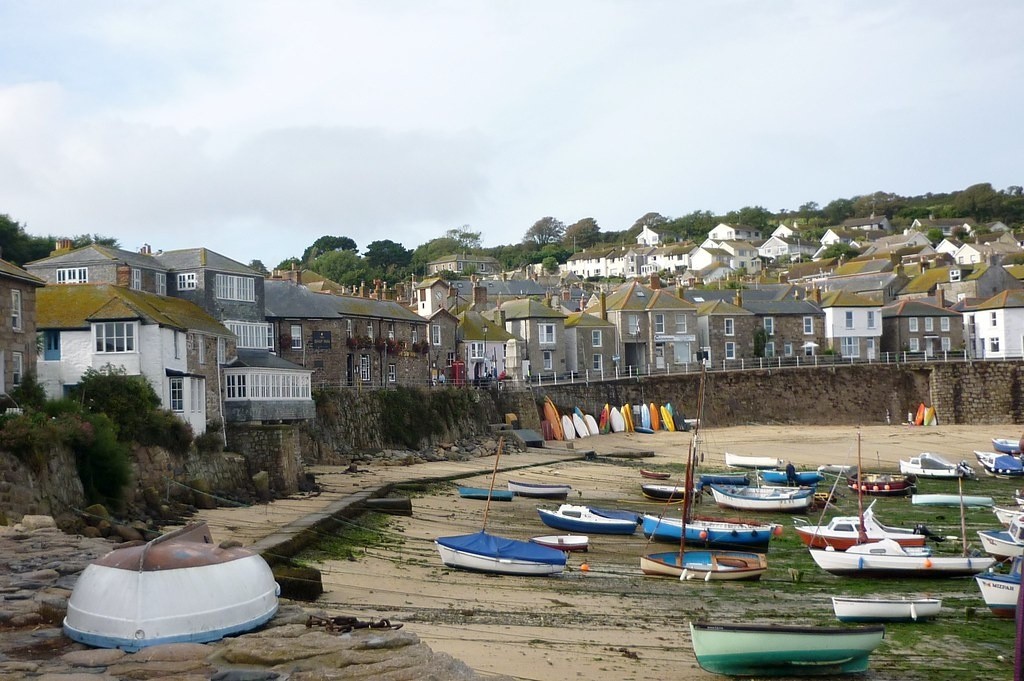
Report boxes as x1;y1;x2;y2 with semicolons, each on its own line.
786;460;797;486
884;407;913;426
433;370;492;387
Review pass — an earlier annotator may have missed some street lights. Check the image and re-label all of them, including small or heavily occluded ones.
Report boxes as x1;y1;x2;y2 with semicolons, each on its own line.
482;323;490;382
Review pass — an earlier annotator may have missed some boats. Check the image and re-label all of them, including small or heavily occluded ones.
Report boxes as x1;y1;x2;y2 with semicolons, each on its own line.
62;520;280;653
433;357;1023;680
